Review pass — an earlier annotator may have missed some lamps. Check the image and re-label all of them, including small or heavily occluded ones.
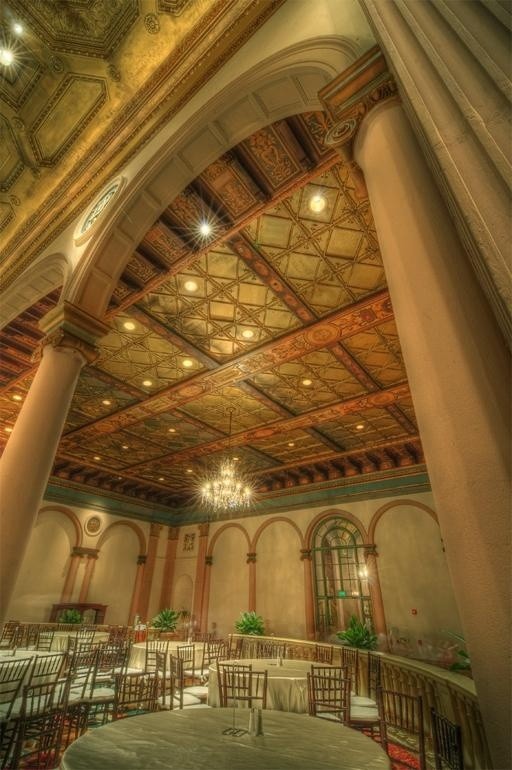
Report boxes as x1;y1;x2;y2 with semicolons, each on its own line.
199;407;254;508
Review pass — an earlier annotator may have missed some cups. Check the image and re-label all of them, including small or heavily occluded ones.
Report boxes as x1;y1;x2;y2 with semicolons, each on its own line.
276;657;283;667
248;708;264;737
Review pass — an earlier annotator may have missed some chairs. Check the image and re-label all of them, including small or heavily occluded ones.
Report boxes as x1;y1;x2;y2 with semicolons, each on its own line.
0;619;465;770
